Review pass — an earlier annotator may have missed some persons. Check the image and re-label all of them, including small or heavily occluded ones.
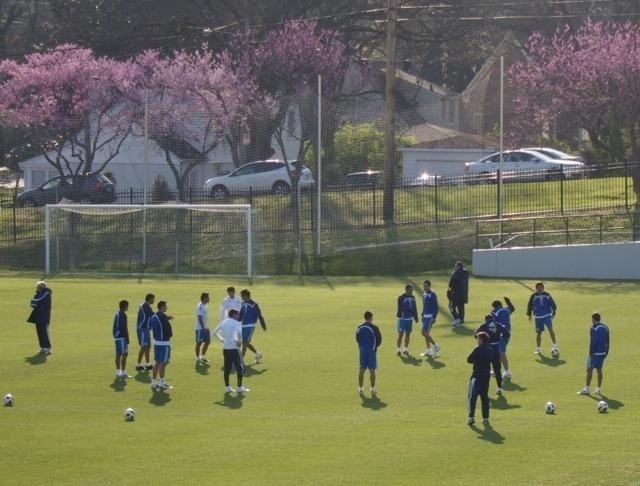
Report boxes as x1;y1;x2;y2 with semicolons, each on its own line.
473;314;511;394
489;296;515;379
466;331;493;426
526;280;558;353
396;283;419;354
576;311;609;395
112;299;131;379
219;286;242;321
421;279;440;356
150;300;173;389
26;280;52;356
194;291;210;363
135;293;156;370
213;307;250;393
239;289;267;364
447;261;468;325
355;310;382;395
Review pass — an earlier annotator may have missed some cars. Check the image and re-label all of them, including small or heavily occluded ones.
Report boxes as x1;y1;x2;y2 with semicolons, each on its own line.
461;147;590;184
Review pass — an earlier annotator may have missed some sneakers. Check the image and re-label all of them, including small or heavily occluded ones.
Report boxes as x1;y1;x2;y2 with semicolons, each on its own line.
359;319;601;425
38;349;51;356
116;353;262;393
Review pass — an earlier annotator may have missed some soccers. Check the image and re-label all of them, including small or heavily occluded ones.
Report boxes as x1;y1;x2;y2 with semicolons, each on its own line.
125;409;136;420
4;394;15;405
551;348;558;355
545;401;555;414
598;401;608;413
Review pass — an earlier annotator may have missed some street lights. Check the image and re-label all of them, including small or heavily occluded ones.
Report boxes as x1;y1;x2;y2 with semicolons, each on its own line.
203;17;252;46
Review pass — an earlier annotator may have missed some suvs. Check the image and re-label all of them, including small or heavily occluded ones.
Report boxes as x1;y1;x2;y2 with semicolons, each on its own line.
205;158;314;202
18;172;118;212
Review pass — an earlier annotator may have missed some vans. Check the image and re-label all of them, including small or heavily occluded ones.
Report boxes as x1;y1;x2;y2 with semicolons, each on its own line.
340;170;381;187
